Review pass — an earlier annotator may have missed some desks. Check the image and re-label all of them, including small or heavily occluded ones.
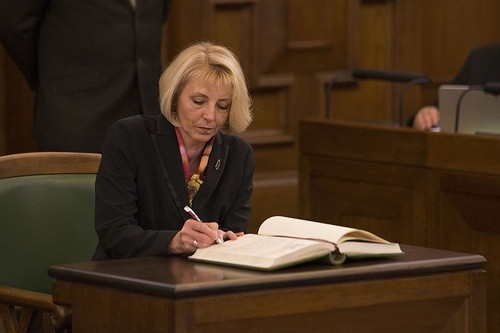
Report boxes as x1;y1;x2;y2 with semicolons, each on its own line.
48;244;488;333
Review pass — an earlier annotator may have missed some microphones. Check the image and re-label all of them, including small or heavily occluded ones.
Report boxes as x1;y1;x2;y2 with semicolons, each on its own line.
454;81;500;132
399;78;434;127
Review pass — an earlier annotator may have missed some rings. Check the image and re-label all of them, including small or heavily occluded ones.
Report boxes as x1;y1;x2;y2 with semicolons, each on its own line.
192;239;199;247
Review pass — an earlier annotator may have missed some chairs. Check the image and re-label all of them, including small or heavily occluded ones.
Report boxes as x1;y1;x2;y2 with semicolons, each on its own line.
0;152;102;333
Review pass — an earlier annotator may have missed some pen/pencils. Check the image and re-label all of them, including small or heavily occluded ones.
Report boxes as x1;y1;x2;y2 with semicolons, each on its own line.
184;205;223;244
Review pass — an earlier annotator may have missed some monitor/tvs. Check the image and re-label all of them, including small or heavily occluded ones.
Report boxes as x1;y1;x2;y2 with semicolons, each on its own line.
439;84;500;135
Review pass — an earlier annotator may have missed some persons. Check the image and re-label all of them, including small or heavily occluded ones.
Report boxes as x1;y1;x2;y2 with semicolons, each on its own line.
0;0;170;154
405;44;500;131
91;40;256;262
